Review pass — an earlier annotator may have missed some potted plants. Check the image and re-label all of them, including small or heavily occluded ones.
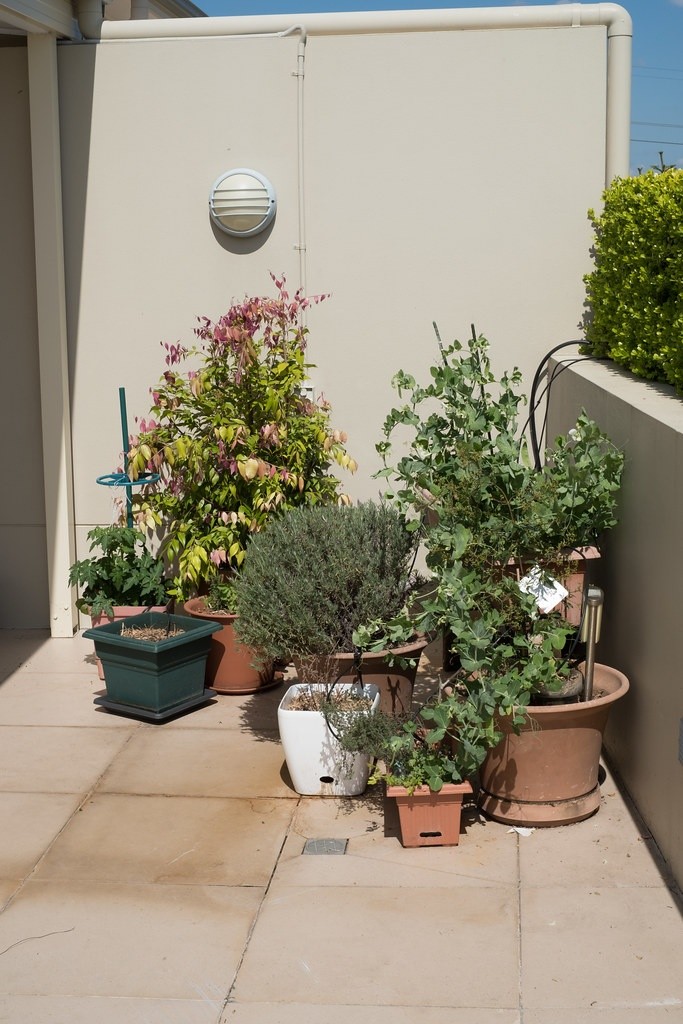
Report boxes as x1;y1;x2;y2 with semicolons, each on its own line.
368;335;627;847
221;495;439;724
278;626;382;796
69;521;190;679
109;268;357;698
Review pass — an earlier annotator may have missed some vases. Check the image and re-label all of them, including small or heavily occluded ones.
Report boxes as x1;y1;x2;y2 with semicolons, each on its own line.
82;610;226;722
441;651;629;828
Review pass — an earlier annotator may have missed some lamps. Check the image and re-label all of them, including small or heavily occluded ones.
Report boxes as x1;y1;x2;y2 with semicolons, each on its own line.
209;168;278;238
